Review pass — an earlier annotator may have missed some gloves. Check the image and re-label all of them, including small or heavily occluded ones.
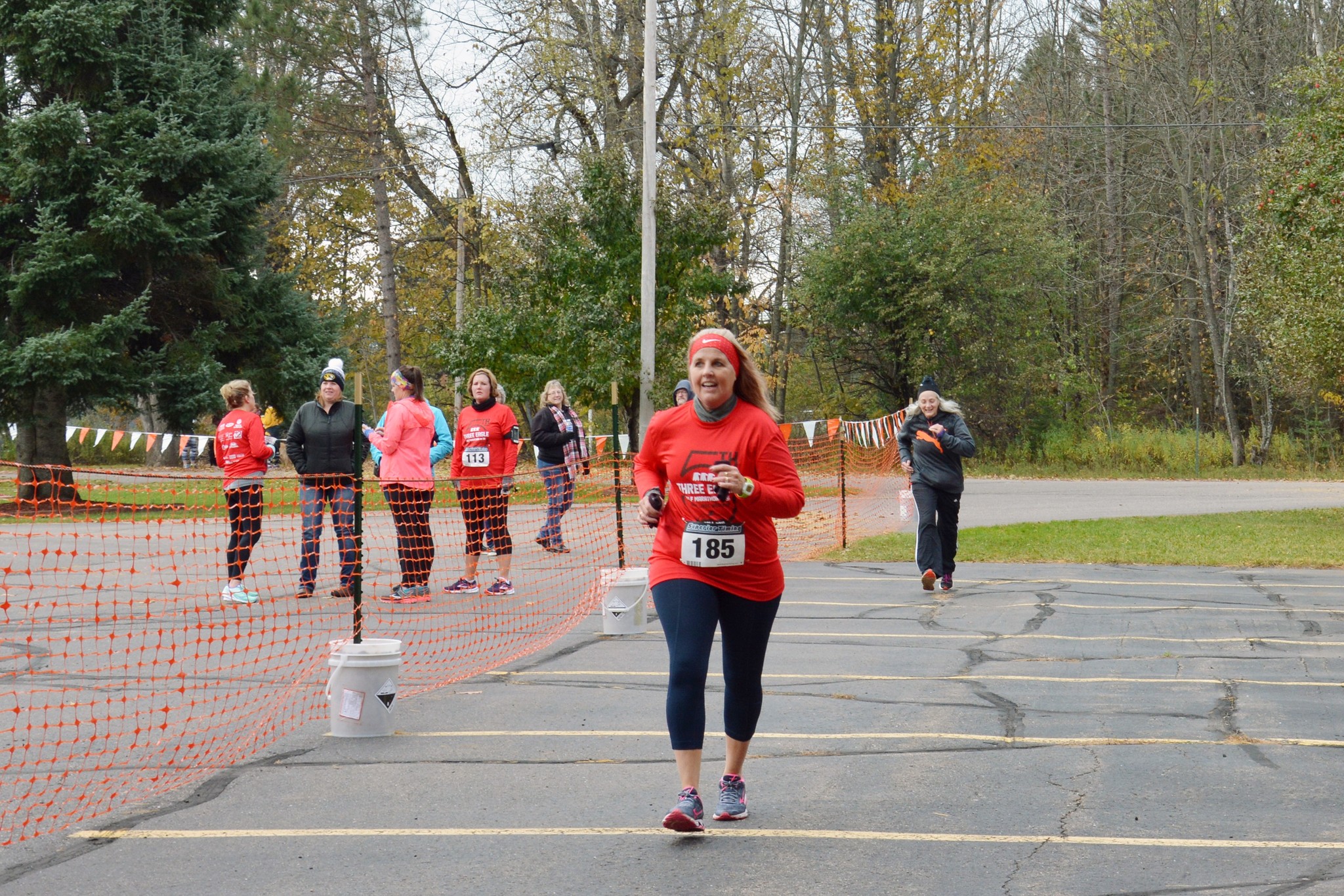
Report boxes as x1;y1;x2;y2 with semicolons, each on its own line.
567;432;575;440
582;466;590;475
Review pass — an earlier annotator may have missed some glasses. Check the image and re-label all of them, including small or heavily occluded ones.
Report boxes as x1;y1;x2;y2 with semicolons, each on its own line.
547;391;562;396
674;390;687;395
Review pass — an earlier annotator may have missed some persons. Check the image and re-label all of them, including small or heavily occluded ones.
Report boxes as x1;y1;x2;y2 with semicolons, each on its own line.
208;410;223;466
673;379;693;405
443;368;515;597
370;392;453;590
213;379;279;604
287;357;370;598
179;419;198;470
252;404;262;416
481;382;505;556
896;379;976;594
260;399;284;466
633;328;804;836
380;365;435;604
530;379;591;554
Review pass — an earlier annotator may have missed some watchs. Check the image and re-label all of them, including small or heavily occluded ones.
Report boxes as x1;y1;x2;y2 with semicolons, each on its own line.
736;475;754;498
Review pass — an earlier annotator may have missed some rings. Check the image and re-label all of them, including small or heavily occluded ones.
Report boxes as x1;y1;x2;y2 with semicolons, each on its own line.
724;471;728;477
725;477;729;483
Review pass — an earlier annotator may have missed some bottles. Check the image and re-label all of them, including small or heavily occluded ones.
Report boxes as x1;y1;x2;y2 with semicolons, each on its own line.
565;419;574;441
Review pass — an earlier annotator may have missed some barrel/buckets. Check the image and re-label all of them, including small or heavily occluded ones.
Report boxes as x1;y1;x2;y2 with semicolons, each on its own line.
899;490;919;522
597;567;649;635
325;639;403;737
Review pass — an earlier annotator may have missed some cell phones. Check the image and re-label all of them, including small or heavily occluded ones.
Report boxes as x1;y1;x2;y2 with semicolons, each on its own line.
513;426;519;443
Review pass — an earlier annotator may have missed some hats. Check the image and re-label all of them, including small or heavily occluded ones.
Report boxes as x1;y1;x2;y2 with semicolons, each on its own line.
917;375;941;399
319;358;345;392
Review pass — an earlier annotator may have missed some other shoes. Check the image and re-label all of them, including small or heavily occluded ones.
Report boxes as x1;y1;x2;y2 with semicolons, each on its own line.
550;544;569;553
486;546;497;556
480;545;487;555
535;537;551;552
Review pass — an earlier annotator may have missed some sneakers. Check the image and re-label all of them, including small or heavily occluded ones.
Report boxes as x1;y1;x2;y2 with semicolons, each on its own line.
712;774;748;820
331;586;355;597
939;573;953;590
379;585;431;604
484;577;515;596
221;584;259;604
921;569;936;591
443;577;478;593
662;786;705;832
296;589;313;598
